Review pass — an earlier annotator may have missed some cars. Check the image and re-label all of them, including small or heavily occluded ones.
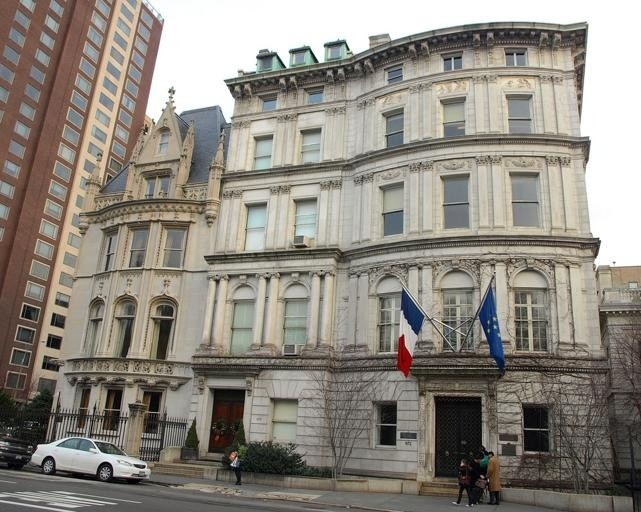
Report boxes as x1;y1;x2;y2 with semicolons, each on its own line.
0;421;151;484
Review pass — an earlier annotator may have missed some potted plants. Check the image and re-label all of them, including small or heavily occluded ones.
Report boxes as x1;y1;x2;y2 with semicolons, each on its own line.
180;416;200;460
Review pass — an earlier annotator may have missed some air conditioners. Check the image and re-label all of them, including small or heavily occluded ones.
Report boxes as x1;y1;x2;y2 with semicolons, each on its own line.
292;235;310;249
282;343;302;357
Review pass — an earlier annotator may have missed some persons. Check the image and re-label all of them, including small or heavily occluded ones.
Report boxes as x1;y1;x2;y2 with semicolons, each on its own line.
228;450;244;485
448;443;503;507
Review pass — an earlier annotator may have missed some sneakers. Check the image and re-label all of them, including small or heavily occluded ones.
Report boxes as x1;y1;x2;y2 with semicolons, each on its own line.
451;499;500;507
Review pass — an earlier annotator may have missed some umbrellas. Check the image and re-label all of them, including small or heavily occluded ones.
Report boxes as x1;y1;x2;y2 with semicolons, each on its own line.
394;286;425;379
476;281;508;375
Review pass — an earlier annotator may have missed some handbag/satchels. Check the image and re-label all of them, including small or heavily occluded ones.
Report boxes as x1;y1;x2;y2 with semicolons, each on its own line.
458;467;468;486
229;453;240;468
